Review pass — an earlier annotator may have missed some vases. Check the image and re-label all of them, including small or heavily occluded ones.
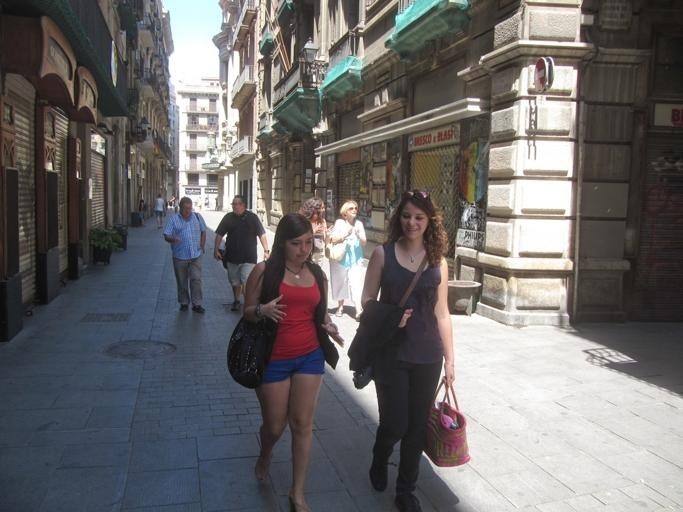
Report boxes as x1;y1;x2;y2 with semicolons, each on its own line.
448;280;481;316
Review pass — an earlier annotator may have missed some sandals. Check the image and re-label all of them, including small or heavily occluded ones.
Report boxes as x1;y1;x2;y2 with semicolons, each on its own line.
289;496;313;512
256;452;271;480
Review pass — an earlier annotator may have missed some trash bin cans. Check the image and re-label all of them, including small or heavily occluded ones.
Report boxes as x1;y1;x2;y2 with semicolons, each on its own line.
131;212;140;227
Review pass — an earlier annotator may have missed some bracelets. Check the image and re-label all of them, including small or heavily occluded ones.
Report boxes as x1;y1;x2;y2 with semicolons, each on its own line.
264;249;269;253
255;303;263;318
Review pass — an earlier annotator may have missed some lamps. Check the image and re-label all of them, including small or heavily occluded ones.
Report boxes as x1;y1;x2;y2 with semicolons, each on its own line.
300;36;319;66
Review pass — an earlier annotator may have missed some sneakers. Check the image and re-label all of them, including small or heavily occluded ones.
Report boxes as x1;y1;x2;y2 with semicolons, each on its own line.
192;305;205;314
394;492;422;512
231;300;241;311
370;459;388;491
180;304;188;312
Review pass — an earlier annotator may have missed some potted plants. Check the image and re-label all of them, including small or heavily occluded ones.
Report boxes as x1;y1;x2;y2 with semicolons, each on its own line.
89;226;125;266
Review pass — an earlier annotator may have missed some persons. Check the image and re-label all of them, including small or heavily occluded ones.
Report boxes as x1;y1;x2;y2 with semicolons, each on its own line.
360;190;455;512
298;196;336;272
243;213;344;512
329;200;367;321
152;192;209;314
214;195;270;311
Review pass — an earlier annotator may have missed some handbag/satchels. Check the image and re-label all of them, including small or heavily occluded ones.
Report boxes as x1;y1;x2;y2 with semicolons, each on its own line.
218;247;227;268
227;315;270;387
426;377;470;467
325;238;348;262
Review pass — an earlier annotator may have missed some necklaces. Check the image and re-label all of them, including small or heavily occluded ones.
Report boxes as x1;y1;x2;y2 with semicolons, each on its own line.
284;263;304;280
405;248;424;264
